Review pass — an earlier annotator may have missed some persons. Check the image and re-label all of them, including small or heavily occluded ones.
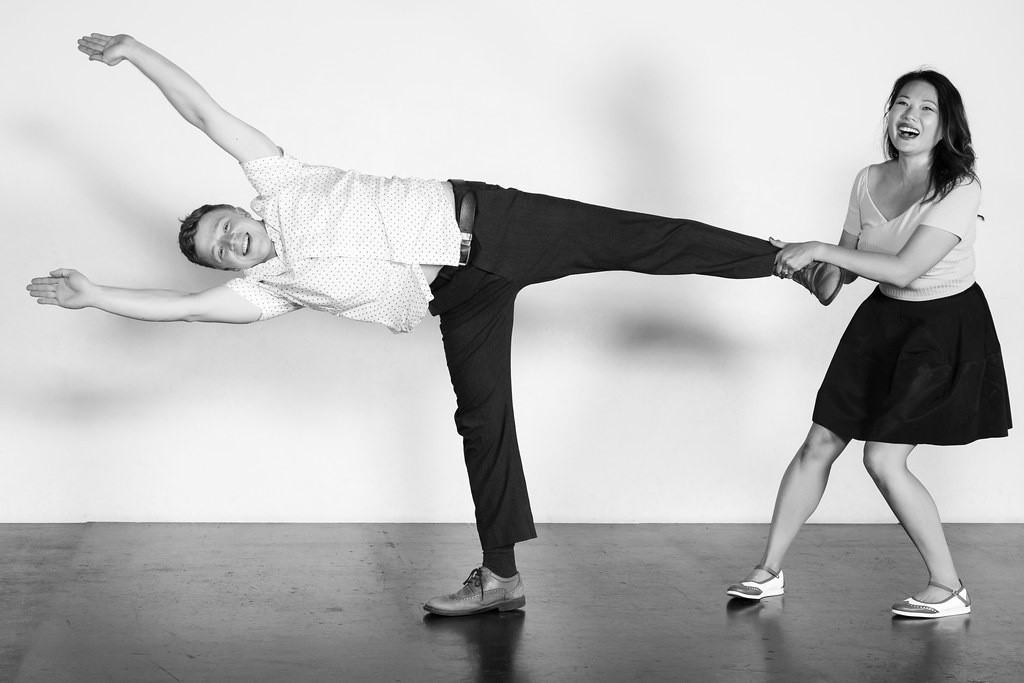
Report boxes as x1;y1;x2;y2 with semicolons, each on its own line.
724;74;1012;620
27;31;845;617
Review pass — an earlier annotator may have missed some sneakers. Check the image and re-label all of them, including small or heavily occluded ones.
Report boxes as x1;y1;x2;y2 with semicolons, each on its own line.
422;566;525;615
790;259;845;306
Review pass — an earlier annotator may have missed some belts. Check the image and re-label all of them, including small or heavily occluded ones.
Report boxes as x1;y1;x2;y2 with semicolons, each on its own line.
428;178;476;291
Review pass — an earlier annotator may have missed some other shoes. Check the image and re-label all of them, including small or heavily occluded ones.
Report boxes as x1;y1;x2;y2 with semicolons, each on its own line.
892;577;971;617
727;563;784;599
